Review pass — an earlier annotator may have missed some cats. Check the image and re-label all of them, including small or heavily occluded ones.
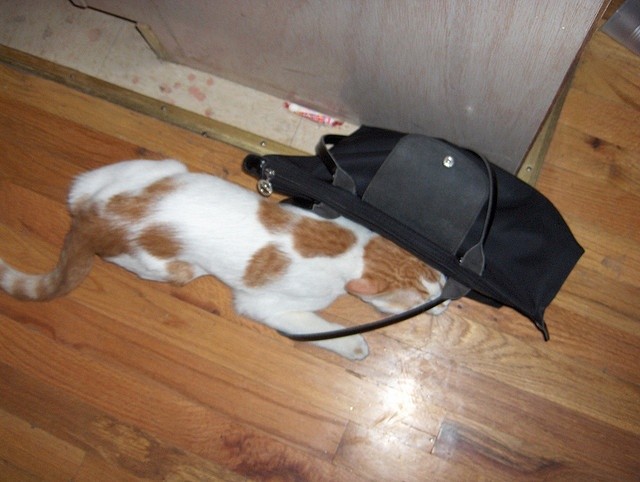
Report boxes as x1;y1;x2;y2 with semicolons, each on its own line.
0;157;453;362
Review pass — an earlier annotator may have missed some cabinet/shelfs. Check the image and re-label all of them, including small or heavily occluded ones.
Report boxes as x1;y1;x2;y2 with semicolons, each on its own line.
70;0;612;177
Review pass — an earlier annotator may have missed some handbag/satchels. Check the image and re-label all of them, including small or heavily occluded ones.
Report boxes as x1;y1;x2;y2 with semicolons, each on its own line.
242;125;584;341
